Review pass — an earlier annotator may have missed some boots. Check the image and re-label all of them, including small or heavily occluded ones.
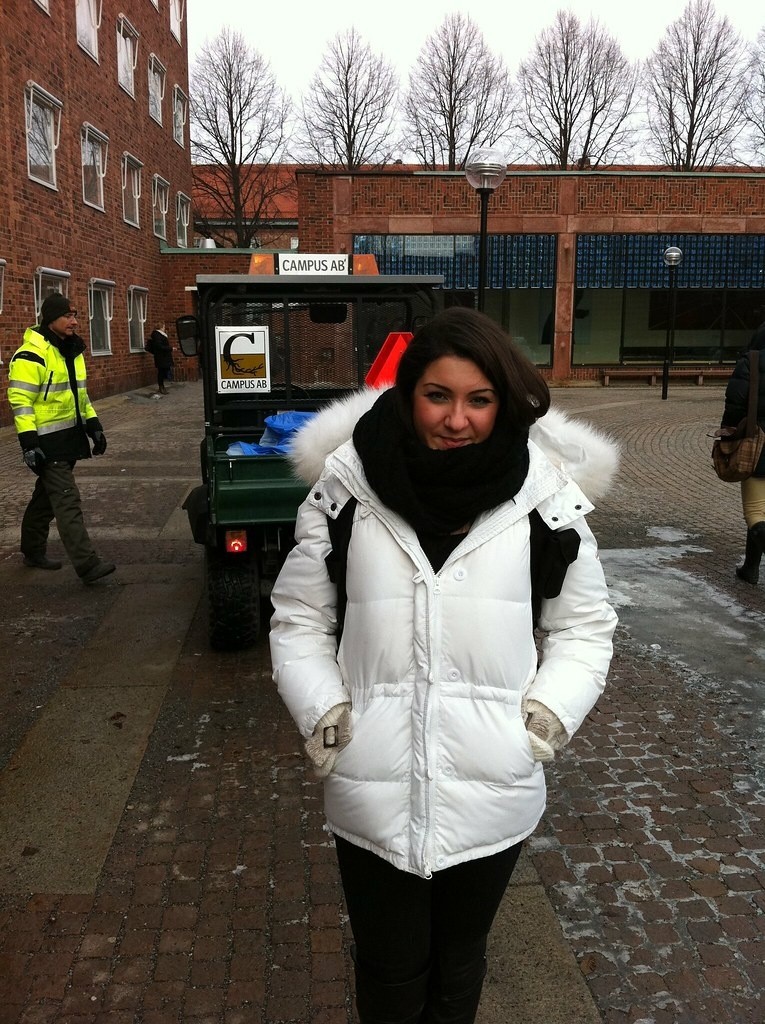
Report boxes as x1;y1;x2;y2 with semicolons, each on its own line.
736;526;763;584
750;521;765;555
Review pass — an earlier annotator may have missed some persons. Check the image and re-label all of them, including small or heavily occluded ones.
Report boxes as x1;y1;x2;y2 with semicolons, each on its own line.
267;306;624;1024
6;293;116;584
151;320;178;395
721;323;765;584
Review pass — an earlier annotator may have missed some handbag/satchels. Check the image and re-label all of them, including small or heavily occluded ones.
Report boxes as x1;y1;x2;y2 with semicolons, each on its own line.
711;419;765;482
145;338;154;354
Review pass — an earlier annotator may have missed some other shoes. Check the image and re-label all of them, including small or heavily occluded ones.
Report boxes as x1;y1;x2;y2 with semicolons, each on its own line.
158;386;169;394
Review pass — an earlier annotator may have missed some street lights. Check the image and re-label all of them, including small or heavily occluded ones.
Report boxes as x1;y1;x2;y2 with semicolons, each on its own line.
660;244;684;399
464;145;509;313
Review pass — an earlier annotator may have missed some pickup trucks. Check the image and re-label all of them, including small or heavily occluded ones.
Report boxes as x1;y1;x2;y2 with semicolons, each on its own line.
172;271;454;653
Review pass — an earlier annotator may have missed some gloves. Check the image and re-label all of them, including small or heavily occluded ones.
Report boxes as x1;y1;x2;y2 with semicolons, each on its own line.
303;702;352;777
522;702;565;761
88;430;107;455
21;447;48;477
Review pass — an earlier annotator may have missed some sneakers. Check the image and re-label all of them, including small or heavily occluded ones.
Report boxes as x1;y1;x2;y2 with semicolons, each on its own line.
23;554;62;570
81;557;116;584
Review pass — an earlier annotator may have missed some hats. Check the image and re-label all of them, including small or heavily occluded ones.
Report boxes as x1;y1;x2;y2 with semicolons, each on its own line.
41;293;77;324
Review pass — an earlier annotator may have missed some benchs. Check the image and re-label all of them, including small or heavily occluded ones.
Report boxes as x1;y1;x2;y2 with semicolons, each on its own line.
602;369;735;386
